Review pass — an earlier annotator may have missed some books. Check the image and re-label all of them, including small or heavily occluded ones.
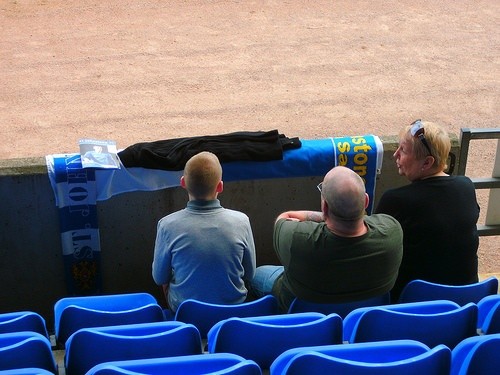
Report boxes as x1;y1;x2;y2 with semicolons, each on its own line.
78;138;121;169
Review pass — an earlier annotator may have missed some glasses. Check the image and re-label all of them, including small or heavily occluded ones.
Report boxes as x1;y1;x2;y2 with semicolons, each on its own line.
409;118;435;156
316;182;324;194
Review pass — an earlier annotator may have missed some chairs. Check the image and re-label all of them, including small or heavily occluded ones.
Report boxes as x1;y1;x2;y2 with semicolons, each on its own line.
1;278;500;375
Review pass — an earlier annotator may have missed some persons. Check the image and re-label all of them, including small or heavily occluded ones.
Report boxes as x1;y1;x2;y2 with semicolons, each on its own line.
84;145;114;165
251;166;403;310
151;151;256;314
375;119;478;298
